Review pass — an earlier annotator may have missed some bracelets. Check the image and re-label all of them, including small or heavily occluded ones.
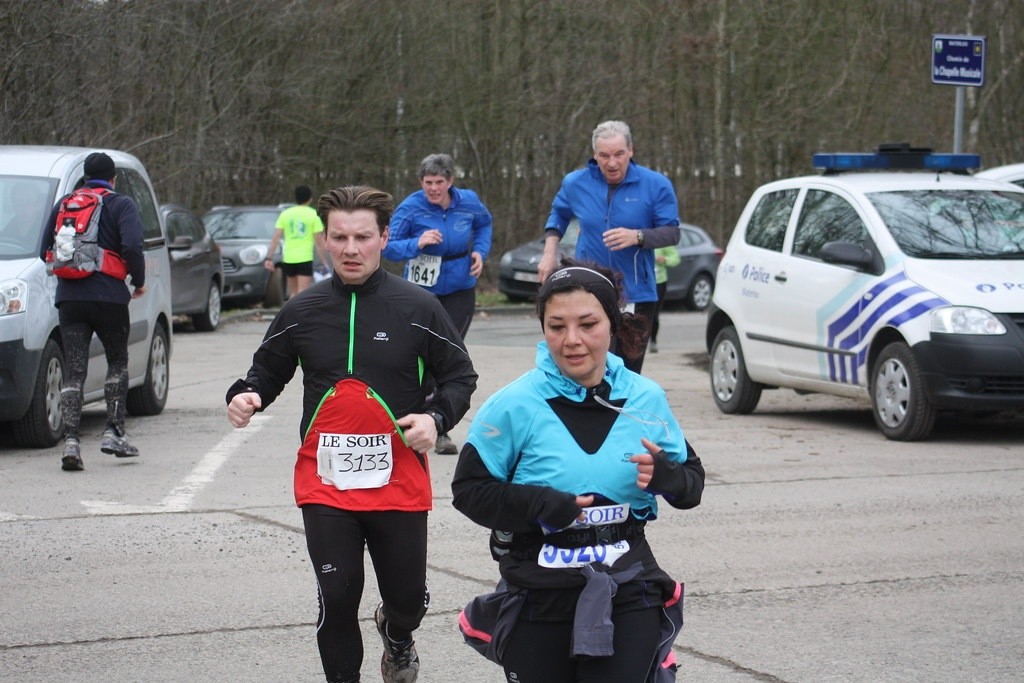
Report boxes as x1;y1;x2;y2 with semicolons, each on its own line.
266;258;273;261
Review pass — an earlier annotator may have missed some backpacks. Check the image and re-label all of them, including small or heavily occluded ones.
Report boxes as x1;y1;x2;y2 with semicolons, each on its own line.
45;187;130;280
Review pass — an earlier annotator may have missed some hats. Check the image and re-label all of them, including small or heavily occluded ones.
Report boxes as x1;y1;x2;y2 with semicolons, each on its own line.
83;152;116;181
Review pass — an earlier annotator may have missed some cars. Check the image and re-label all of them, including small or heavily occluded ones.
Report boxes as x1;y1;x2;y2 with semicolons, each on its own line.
158;203;226;334
497;222;726;313
200;202;332;311
704;141;1023;442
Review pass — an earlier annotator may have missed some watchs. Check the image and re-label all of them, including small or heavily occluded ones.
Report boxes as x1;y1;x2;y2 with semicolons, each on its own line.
427;410;445;434
637;229;644;246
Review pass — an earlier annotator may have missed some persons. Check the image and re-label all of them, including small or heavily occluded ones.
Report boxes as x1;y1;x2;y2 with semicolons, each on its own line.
538;121;681;375
650;246;680;352
40;152;147;470
452;259;705;683
264;186;330;300
225;187;478;683
383;154;492;453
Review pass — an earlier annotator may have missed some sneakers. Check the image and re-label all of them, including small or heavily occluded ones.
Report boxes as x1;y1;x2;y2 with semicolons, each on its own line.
99;427;139;457
374;600;420;683
434;433;458;455
61;439;85;471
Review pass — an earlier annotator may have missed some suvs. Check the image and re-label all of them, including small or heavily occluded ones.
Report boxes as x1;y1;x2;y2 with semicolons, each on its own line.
0;143;173;451
